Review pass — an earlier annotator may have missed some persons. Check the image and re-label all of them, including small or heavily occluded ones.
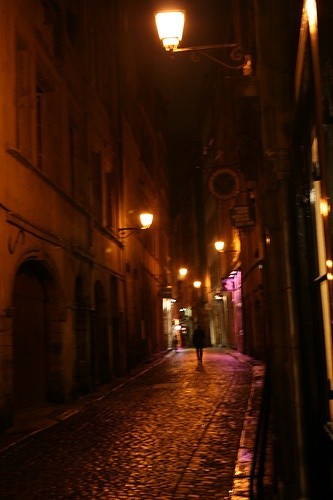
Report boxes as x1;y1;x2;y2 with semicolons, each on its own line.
193;325;205;362
